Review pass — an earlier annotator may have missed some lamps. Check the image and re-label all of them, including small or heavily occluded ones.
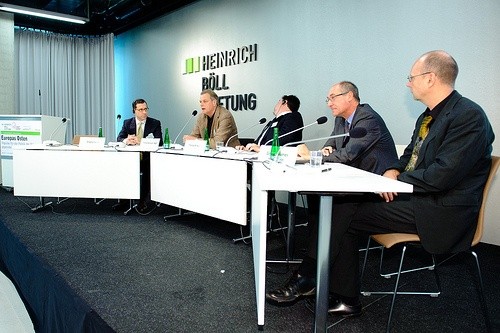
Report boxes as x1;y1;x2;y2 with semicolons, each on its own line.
0;3;89;24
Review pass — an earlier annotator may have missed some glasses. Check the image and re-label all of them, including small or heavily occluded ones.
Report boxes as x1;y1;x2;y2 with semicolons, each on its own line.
408;71;431;82
325;91;349;102
136;108;149;112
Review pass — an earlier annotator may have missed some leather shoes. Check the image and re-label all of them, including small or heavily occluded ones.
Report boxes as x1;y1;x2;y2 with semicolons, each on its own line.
266;270;317;308
305;290;363;318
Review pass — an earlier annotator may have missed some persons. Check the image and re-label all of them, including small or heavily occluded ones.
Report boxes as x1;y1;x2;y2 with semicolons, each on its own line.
266;50;496;317
117;99;163;146
295;81;398;257
235;95;305;151
183;90;241;148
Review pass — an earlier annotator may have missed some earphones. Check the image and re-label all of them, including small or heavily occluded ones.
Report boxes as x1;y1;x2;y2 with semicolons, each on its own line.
283;101;286;104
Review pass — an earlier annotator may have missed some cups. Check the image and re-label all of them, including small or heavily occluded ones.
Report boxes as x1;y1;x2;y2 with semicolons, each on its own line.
216;142;224;151
309;150;323;168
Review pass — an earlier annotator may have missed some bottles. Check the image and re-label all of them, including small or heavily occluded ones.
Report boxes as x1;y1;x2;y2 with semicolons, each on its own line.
163;128;170;149
98;128;103;137
270;128;281;160
203;128;210;151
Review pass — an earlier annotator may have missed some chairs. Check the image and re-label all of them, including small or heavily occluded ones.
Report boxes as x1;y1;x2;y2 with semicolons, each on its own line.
267;144;500;333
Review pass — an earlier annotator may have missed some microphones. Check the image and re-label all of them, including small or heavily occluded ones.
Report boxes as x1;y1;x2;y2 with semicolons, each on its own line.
170;110;197;149
219;118;267;151
262;128;368;171
109;114;121;146
248;116;328;159
43;118;67;146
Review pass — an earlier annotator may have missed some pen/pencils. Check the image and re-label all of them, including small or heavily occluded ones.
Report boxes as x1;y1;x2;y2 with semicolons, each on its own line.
322;168;331;172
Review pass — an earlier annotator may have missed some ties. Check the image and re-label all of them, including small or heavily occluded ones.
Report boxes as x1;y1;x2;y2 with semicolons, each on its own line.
342;122;350;141
137;122;144;160
404;116;432;171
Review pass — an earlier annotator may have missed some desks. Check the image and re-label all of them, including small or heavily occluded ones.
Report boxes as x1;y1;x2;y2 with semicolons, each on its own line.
150;147;259;241
11;145;160;216
247;153;413;332
